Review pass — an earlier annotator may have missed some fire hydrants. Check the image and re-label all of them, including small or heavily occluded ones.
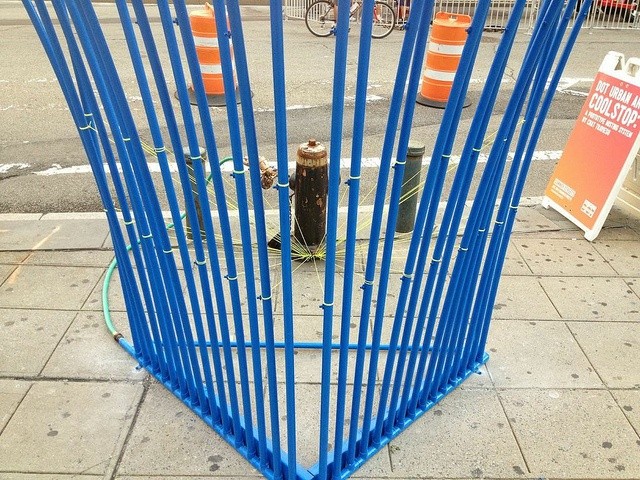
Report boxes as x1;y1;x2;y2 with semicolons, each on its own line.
268;140;341;263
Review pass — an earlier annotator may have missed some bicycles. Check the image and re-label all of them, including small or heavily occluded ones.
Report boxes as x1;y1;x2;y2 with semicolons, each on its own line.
305;0;395;38
